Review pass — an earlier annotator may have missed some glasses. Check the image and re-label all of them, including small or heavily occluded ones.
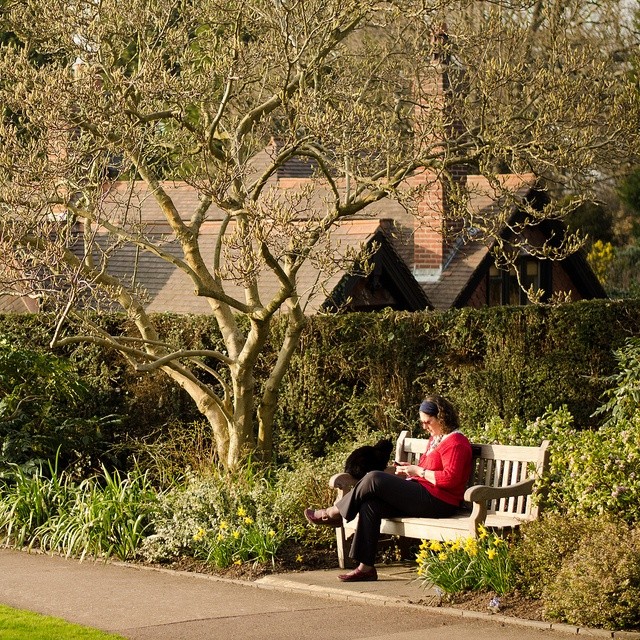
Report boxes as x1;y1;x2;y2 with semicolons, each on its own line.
419;420;438;427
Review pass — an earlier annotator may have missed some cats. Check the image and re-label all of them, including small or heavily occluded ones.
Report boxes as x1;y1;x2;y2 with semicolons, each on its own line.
344;435;394;482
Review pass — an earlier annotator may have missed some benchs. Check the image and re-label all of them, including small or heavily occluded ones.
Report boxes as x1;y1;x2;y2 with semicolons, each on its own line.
327;429;553;570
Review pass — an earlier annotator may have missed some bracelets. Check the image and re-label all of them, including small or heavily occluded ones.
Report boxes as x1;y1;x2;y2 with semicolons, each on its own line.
421;469;426;478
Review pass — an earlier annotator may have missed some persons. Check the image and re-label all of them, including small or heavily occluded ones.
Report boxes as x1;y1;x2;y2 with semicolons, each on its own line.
304;394;473;583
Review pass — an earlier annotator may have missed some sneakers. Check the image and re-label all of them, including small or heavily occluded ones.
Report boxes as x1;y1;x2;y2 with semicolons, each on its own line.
336;565;377;581
304;507;343;528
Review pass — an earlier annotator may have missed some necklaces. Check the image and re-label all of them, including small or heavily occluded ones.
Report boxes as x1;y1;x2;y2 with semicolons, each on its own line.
430;434;443;448
